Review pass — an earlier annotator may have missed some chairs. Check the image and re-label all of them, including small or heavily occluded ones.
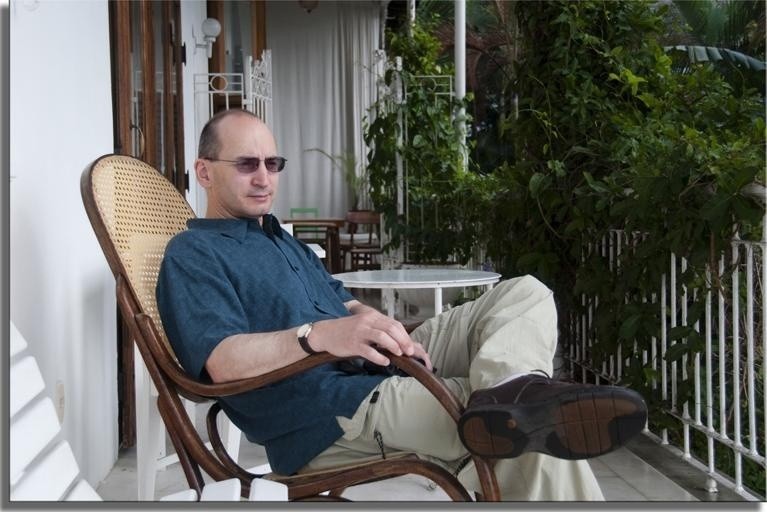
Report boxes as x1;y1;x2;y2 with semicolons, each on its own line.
339;209;376;257
289;208;320;238
345;212;382;269
80;154;502;502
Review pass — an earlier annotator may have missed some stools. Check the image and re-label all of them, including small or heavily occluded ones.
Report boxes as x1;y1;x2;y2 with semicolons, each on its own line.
289;221;338;274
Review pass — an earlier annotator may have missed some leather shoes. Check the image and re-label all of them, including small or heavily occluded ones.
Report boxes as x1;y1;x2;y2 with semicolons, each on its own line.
458;371;649;462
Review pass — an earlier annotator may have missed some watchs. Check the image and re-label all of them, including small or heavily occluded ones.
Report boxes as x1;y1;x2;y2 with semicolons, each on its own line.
297;320;318;357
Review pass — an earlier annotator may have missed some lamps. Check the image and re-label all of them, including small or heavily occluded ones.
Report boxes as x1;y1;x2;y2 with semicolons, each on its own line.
299;0;319;14
191;18;221;60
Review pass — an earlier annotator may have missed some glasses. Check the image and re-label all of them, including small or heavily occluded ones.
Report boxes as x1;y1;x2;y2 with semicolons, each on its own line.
204;155;289;175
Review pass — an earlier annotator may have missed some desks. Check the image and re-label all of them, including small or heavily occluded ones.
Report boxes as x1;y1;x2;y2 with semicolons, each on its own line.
329;269;502;319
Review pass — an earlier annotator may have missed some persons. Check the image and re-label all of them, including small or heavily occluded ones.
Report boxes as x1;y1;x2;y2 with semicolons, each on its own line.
152;108;650;502
